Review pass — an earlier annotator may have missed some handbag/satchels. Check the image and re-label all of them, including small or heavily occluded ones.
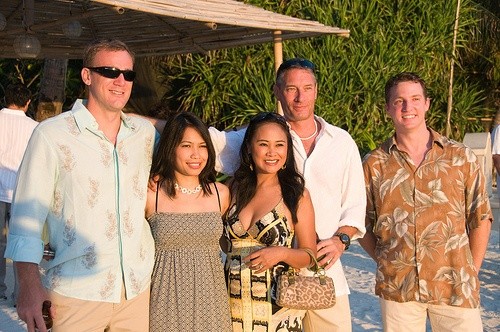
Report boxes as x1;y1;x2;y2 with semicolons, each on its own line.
276;247;335;310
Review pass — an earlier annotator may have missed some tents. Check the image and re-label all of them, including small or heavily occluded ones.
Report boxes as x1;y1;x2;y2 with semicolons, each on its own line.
0;0;351;123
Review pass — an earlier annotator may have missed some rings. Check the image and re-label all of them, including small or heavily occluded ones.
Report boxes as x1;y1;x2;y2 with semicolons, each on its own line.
259;263;263;269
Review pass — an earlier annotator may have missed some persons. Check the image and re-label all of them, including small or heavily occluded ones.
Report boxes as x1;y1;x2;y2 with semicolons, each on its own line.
144;112;232;332
147;112;317;332
358;72;495;332
0;82;40;307
124;58;367;332
3;39;161;332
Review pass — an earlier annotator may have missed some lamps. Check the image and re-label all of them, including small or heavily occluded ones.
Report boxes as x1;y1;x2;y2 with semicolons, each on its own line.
0;0;81;58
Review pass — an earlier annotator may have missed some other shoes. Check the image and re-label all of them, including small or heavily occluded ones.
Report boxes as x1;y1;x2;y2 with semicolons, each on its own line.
0;295;7;300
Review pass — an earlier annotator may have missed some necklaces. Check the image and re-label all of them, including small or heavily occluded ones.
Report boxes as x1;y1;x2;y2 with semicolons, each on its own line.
300;119;317;140
174;183;202;194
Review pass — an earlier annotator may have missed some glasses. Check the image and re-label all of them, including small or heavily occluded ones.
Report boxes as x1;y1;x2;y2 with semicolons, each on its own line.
250;112;286;127
277;59;315;78
86;67;136;81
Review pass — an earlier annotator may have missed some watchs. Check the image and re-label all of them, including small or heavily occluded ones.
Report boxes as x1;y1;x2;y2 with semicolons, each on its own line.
333;232;350;250
